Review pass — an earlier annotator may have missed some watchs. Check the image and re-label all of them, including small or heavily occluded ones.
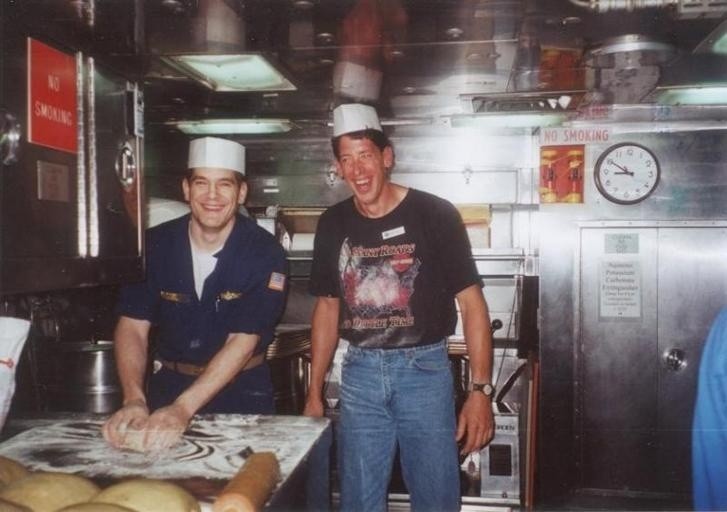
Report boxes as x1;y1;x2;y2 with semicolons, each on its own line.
468;382;495;398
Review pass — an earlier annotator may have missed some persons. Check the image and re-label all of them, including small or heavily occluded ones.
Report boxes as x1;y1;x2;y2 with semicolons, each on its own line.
300;129;496;511
99;166;291;454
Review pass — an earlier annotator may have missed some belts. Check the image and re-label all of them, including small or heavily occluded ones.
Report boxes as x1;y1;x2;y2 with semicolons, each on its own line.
161;353;264;378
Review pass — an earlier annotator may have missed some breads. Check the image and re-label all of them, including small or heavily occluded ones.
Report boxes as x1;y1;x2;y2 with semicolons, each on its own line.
0;453;202;512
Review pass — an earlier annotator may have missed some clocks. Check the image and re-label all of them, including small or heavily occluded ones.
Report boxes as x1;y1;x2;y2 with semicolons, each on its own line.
595;143;662;205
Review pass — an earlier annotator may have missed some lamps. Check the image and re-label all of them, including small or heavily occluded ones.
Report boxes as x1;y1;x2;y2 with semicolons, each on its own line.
162;118;302;135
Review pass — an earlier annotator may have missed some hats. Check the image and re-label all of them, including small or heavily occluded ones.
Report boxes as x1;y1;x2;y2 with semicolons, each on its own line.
333;62;383;101
333;103;383;139
194;1;244;45
187;137;246;175
